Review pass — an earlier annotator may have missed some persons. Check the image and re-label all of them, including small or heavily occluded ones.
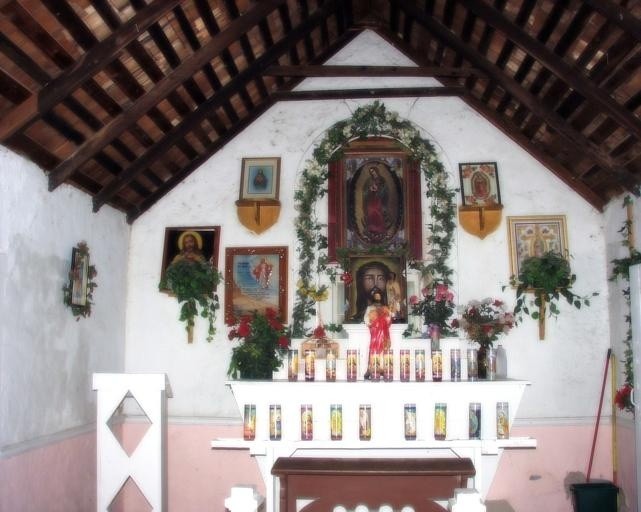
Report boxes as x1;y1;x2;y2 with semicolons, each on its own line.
362;286;393;377
363;179;386;233
352;261;396;319
383;270;401;311
252;259;273;288
168;233;206;272
253;168;269;188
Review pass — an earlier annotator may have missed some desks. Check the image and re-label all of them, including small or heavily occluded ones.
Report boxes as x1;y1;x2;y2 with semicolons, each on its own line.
271;457;476;512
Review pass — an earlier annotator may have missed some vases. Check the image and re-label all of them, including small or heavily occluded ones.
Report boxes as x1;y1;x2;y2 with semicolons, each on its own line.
403;403;416;440
244;404;256;441
428;320;440;351
469;403;481;440
287;345;498;382
300;404;313;441
240;367;272;381
435;403;447;440
497;402;509;439
330;404;343;440
359;404;371;441
270;405;282;441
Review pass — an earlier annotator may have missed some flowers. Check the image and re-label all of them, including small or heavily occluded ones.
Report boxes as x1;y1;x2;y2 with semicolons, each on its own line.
410;282;458;335
225;306;288;380
450;296;515;349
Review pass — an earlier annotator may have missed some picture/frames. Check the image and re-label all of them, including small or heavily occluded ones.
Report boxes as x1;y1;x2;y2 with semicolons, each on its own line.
158;225;221;294
327;138;423;265
508;217;572;291
239;156;281;202
345;250;407;324
70;247;89;307
459;161;502;208
224;246;288;324
